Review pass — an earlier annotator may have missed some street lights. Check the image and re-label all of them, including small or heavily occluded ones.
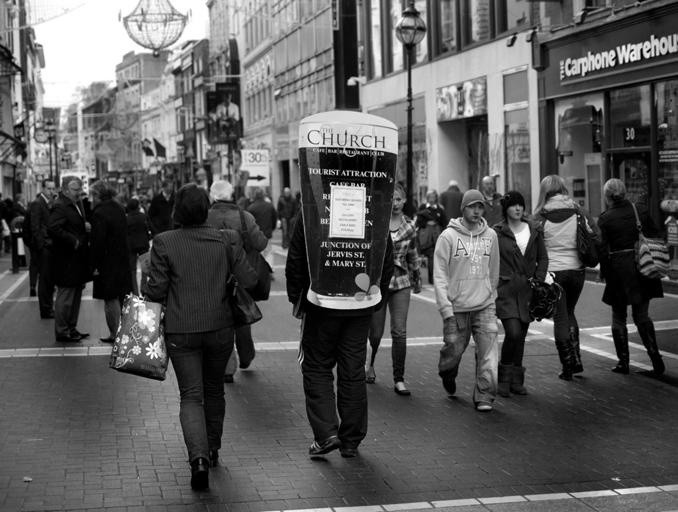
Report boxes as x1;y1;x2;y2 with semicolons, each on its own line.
44;120;57;180
391;1;430;217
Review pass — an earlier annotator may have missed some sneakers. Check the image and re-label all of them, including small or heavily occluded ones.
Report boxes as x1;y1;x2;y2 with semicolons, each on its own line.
190;459;209;491
309;433;342;455
224;348;256;383
475;401;493;412
395;382;410;396
444;374;457;396
209;446;218;468
342;446;358;457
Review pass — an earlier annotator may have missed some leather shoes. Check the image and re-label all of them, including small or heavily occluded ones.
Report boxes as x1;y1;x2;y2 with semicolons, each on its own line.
30;287;55;319
56;329;89;342
100;335;116;343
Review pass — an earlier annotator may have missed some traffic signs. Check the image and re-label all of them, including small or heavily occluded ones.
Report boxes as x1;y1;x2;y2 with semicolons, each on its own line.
239;149;270;185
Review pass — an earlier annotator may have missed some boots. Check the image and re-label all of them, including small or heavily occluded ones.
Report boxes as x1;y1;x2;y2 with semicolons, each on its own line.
554;324;584;380
498;362;527;397
612;326;630;374
639;322;665;377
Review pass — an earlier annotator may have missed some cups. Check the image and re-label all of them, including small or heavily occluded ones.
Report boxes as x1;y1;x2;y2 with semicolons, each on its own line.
296;121;403;310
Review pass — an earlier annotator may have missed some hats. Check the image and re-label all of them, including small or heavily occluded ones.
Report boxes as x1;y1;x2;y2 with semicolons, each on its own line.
173;184;208;225
209;179;234;203
461;188;487;211
501;190;525;214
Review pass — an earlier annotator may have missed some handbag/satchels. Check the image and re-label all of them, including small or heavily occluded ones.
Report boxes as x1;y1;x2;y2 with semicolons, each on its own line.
417;222;441;251
109;294;168;381
248;246;274;301
528;279;563;321
634;228;671;279
577;224;605;268
227;273;262;329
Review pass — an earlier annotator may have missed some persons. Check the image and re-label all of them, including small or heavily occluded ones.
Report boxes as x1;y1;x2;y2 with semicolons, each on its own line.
440;178;468;223
596;177;672;374
283;195;400;458
489;187;550;398
365;180;410;396
479;175;508;232
0;166;308;384
524;173;599;379
436;188;500;409
143;181;260;492
417;189;447;284
213;91;239;135
